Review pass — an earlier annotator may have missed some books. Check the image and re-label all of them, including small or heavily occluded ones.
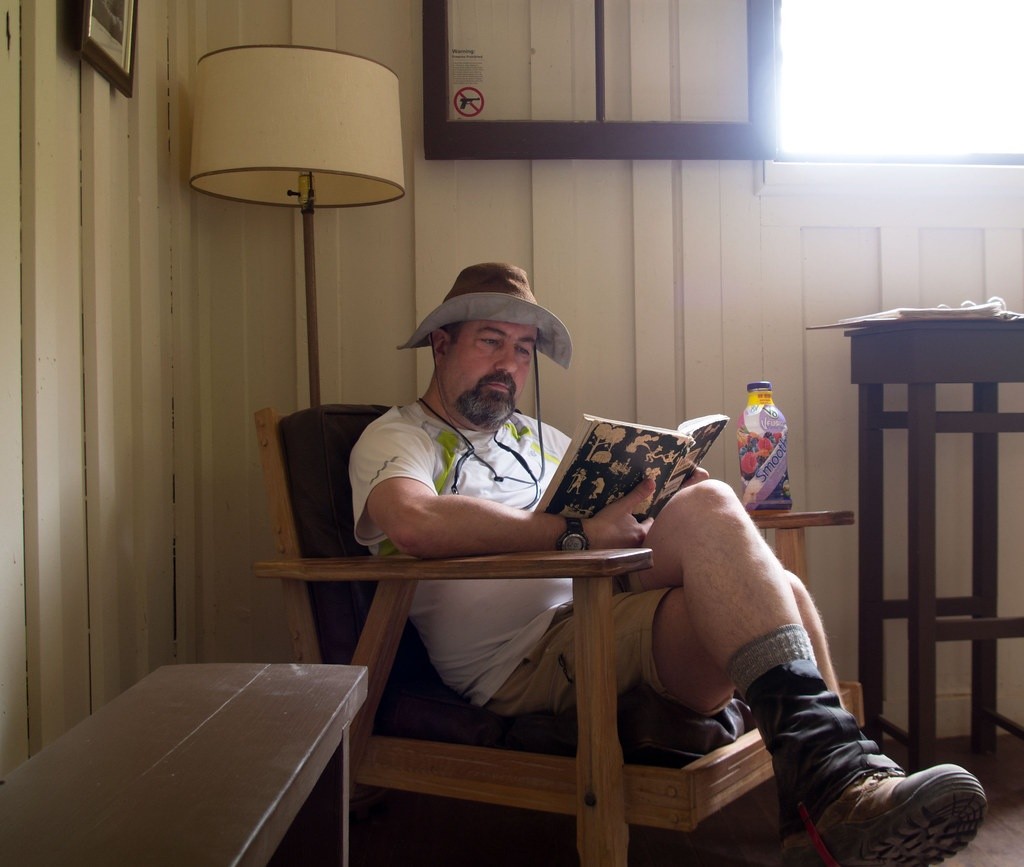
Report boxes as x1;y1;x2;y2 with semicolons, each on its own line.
532;412;730;517
806;298;1024;323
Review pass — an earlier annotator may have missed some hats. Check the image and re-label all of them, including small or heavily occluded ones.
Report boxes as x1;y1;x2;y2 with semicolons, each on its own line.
394;263;572;369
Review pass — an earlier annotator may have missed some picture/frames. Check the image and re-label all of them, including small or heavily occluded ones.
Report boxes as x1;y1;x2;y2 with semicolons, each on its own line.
78;0;139;99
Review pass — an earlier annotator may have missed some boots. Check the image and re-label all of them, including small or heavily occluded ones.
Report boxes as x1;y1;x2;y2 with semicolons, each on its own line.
726;623;987;867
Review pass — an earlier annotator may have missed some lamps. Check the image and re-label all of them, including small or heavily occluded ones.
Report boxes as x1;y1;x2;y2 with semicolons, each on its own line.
186;43;406;405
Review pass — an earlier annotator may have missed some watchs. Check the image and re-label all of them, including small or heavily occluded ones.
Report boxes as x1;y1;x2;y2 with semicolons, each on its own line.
556;518;589;552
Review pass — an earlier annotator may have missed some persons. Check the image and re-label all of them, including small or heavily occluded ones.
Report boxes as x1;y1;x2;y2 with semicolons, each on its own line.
344;259;987;867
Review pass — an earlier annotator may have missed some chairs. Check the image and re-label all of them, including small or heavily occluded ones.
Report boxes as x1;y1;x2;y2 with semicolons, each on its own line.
249;403;870;867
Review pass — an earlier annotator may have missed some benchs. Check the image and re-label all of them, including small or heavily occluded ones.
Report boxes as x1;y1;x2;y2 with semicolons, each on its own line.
0;662;370;867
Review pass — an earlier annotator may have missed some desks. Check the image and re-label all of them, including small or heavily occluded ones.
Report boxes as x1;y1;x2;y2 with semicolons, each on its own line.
842;320;1024;772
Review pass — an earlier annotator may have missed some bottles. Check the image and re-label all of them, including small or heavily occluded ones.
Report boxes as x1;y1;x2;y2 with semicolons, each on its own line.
738;382;792;516
